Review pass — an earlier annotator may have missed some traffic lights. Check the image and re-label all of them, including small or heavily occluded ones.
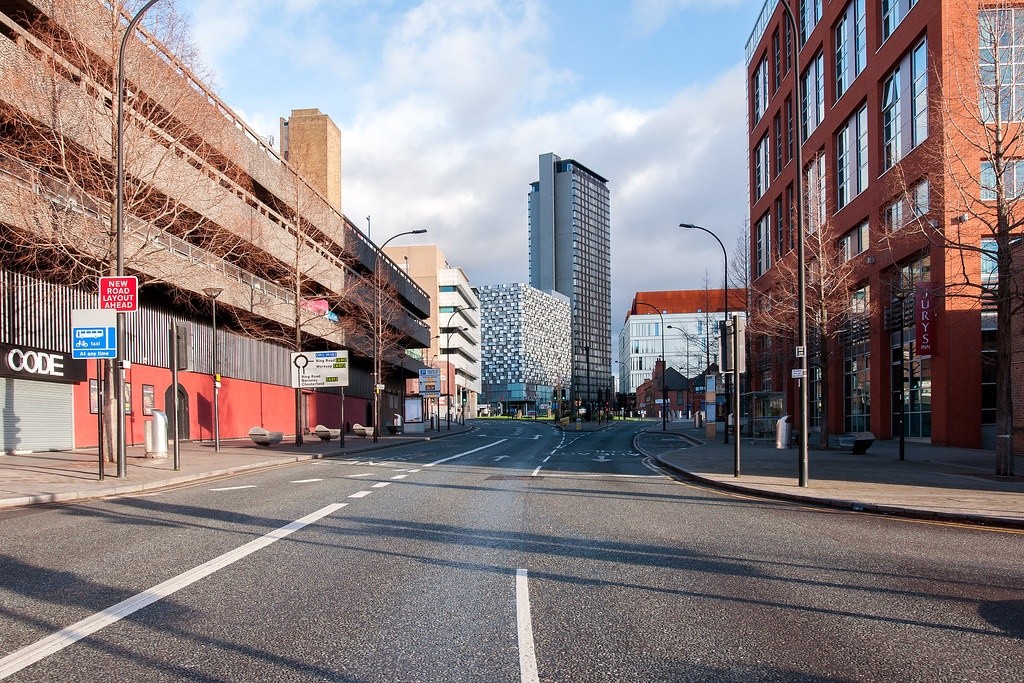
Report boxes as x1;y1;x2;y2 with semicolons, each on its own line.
561;389;566;400
606;402;609;412
553;390;557;400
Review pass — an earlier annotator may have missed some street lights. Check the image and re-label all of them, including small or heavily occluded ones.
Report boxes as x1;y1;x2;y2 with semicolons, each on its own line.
374;228;428;443
615;361;630;418
634;302;666;431
667;325;689;420
679;223;729;444
447;307;478;430
202;287;224;452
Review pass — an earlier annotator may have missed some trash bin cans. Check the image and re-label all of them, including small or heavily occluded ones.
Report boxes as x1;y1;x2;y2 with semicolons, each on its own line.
694;410;702;428
774;415;793;450
393;413;402;435
150;408;168;452
430;413;438;430
